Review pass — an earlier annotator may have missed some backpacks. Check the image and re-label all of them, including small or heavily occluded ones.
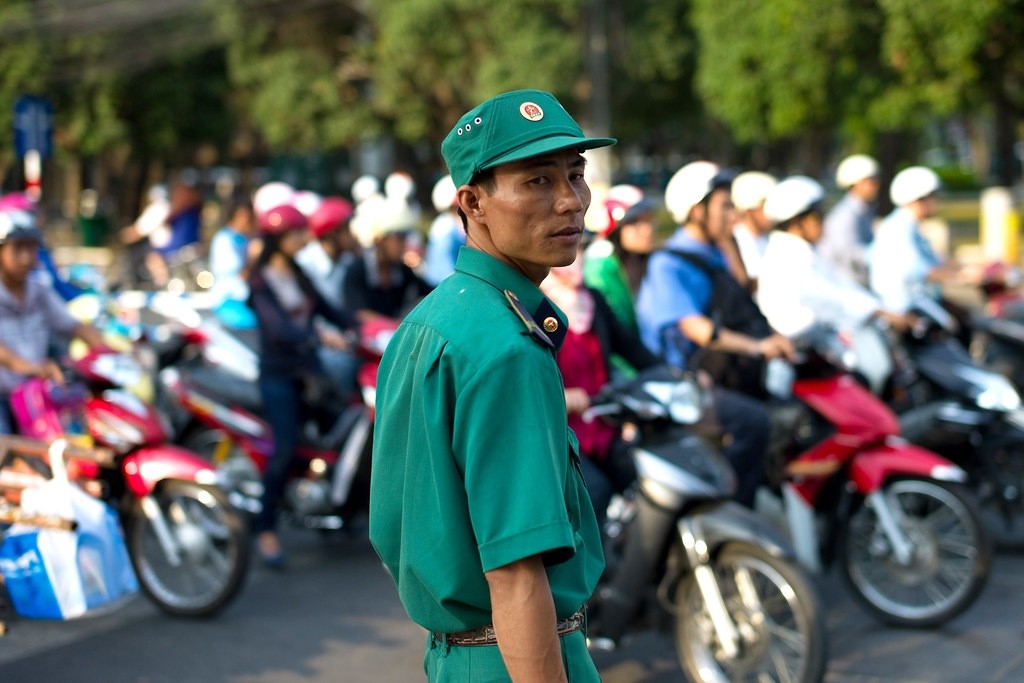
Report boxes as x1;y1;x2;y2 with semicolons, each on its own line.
646;247;774;386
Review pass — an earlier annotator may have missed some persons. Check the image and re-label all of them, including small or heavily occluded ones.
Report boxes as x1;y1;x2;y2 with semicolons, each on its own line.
0;153;1007;570
367;89;620;683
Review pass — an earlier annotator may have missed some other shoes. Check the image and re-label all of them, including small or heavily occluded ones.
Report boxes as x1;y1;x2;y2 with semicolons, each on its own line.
255;536;285;570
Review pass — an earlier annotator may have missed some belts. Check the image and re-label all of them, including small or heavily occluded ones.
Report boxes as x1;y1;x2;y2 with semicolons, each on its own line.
434;602;587;648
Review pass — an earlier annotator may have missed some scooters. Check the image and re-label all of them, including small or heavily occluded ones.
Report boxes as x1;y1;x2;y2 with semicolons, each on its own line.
563;277;831;683
755;262;1024;633
25;275;409;626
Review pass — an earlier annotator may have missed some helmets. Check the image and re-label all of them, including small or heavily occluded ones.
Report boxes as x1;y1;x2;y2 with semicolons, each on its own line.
351;176;381;201
833;153;881;190
665;162;734;224
889;166;942;204
252;181;295;216
257;206;310;236
730;170;782;210
763;175;826;222
305;198;357;237
0;206;39;241
0;192;37;211
431;174;460;213
386;173;416;201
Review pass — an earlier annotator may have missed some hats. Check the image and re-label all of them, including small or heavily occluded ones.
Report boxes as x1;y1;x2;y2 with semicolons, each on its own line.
440;89;618;193
